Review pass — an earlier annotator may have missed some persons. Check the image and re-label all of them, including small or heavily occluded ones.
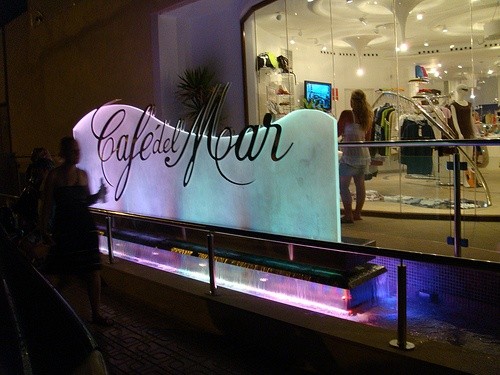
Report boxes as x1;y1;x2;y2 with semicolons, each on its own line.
1;155;49;246
335;90;375;224
449;84;476;188
36;138;117;328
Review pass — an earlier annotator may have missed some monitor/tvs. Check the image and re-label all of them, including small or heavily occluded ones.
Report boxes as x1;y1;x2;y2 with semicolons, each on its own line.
304;80;332;110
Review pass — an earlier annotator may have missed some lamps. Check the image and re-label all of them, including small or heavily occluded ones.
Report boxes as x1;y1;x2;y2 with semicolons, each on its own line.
290;29;327;50
424;23;494;78
276;11;282;21
